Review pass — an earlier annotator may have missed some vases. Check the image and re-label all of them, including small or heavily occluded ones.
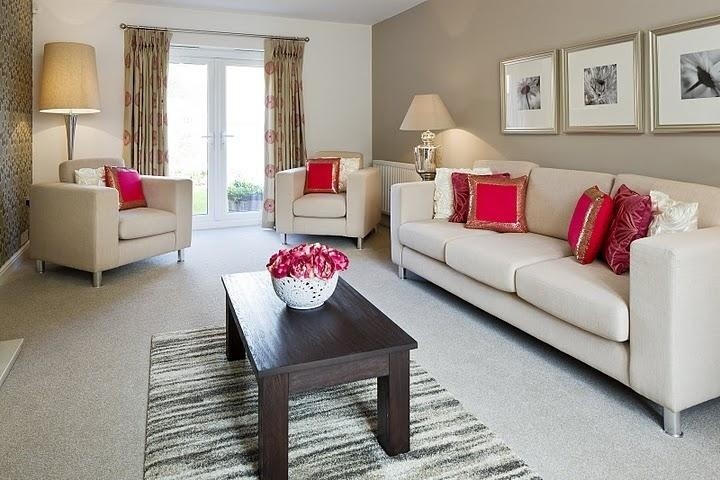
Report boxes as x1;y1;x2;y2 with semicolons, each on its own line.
267;272;341;311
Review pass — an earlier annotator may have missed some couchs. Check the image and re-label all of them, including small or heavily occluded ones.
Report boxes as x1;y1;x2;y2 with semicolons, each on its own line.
274;148;384;250
27;152;195;290
391;156;720;438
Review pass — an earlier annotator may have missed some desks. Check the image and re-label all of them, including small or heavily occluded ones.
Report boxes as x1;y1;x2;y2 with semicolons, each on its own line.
218;266;420;479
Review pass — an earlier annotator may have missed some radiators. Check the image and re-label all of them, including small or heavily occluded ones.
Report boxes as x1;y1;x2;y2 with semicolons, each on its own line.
372;158;425;218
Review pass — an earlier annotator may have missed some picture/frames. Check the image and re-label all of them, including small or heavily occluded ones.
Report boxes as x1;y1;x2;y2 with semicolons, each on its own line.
497;48;561;137
558;29;648;140
643;12;720;138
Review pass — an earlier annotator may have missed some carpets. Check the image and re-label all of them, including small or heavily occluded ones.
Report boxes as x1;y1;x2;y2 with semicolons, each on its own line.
140;311;548;480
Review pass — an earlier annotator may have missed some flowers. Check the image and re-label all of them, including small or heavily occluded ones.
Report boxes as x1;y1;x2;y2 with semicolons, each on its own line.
263;244;350;277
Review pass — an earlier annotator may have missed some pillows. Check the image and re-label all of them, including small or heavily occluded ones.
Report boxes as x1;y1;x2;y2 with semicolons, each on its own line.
304;155;340;194
566;187;615;266
326;155;361;192
465;174;530;236
73;166;109;189
450;170;511;223
640;187;701;237
430;166;494;220
606;181;652;276
104;163;147;211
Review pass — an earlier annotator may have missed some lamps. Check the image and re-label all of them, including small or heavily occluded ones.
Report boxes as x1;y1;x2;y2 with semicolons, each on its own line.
397;93;457;182
35;40;103;165
